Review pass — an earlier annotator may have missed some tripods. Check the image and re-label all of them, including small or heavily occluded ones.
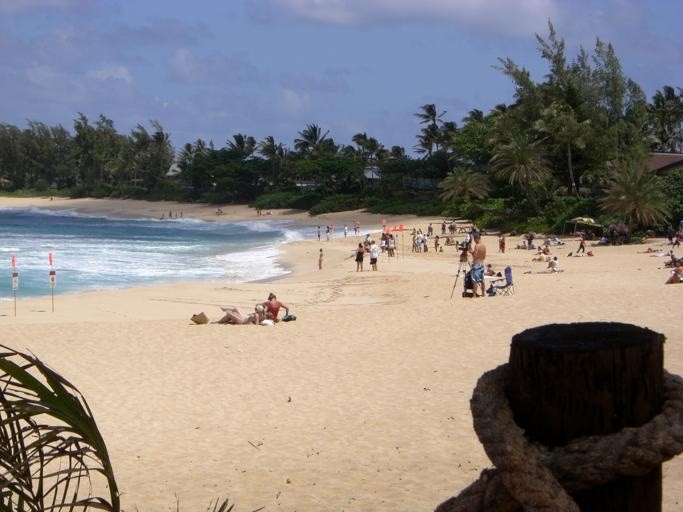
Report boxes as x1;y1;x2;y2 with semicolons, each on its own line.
451;256;471;299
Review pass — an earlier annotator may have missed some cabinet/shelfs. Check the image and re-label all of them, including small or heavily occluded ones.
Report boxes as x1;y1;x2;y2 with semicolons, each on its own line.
450;238;477;299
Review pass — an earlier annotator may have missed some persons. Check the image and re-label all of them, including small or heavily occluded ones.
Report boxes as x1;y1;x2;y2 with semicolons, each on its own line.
215;304;267;326
468;233;485;298
317;225;320;241
260;292;289;323
326;218;586;276
638;222;682;283
318;248;322;269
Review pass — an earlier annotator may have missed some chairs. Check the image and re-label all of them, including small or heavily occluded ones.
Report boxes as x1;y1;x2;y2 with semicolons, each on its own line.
493;266;514;297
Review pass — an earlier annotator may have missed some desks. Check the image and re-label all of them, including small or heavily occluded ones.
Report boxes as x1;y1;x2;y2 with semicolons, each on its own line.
483;274;505;294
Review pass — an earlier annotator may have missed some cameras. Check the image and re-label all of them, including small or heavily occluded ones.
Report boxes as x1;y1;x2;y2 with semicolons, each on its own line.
459;247;468;251
